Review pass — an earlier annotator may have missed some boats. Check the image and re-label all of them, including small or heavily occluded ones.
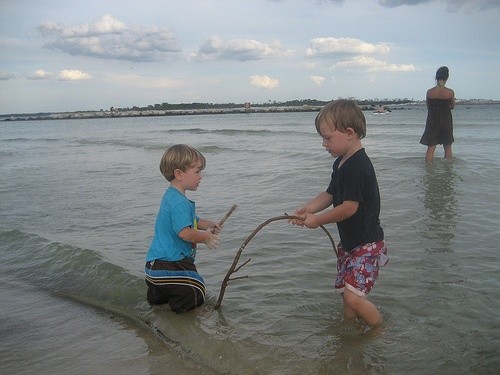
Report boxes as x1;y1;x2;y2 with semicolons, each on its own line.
374;110;389;116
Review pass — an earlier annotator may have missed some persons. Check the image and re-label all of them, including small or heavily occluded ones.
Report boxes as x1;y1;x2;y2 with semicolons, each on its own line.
144;144;223;316
419;66;456;162
287;98;390;326
